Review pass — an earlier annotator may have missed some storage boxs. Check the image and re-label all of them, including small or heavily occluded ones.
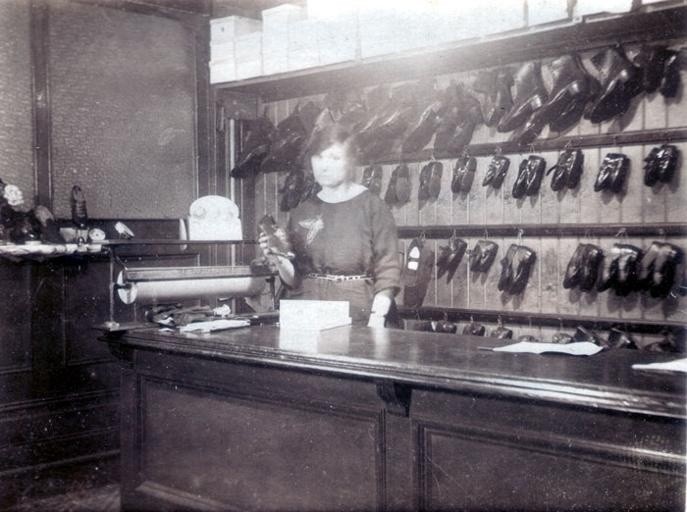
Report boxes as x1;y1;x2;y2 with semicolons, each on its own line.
205;0;313;88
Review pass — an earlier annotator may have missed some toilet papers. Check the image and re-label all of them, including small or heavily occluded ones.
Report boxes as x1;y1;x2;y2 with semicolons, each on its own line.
115;264;278;304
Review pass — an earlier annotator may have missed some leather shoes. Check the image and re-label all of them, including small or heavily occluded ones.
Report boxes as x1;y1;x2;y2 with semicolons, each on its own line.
228;117;322;211
40;218;61;242
402;239;536;299
353;93;482;206
563;241;680;292
71;185;87;229
434;321;632;349
482;146;678;198
21;223;38;240
258;216;298;261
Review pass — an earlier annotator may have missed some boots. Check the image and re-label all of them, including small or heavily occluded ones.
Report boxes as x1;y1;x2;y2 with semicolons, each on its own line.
483;45;680;145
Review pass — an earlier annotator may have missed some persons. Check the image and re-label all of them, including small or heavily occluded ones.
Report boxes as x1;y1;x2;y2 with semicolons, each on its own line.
257;125;406;329
